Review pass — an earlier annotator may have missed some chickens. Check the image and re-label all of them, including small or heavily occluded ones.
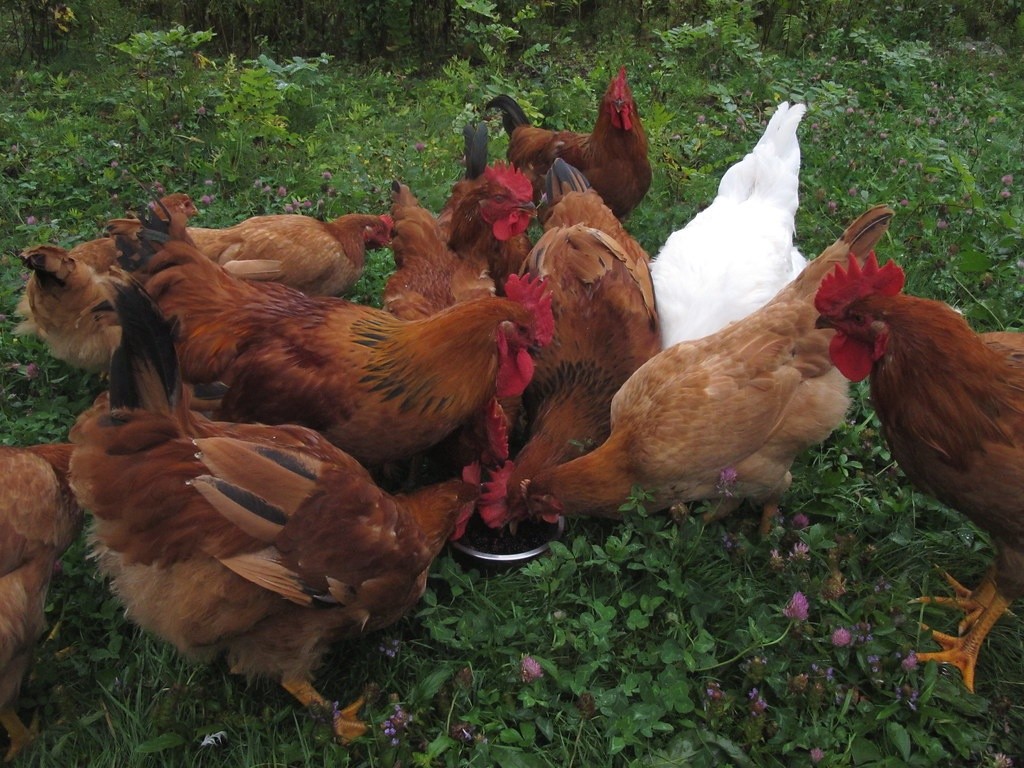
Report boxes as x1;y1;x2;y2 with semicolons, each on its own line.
0;66;1023;763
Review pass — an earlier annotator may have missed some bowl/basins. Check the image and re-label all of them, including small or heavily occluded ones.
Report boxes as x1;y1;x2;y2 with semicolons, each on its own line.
456;510;566;566
